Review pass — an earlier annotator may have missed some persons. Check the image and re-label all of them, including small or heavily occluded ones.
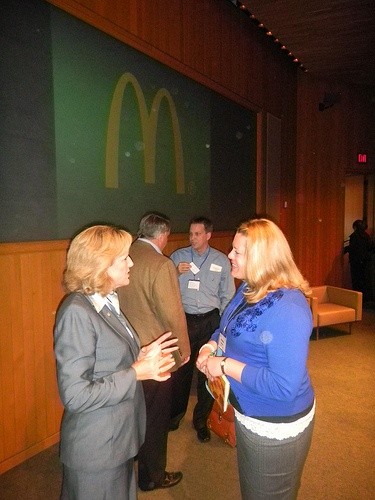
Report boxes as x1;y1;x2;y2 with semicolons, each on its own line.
114;211;192;493
53;224;179;500
169;218;236;443
194;218;316;500
345;220;375;309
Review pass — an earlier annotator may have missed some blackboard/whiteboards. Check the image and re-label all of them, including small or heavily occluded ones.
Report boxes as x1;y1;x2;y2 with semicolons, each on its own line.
0;0;263;255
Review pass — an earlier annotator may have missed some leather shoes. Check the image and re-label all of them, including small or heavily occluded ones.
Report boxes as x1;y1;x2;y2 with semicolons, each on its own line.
144;472;183;491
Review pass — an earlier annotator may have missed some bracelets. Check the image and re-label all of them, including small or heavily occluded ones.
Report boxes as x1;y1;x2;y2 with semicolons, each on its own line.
198;343;215;354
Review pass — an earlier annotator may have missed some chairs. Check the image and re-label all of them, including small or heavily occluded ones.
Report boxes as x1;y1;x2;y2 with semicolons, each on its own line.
306;285;363;341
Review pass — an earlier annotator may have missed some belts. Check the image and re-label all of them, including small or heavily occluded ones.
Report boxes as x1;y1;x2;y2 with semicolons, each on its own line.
185;310;215;320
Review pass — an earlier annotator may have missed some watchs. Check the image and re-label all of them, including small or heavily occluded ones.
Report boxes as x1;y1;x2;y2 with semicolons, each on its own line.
220;356;229;374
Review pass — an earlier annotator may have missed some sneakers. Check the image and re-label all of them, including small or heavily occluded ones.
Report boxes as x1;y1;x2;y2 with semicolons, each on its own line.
194;420;210;441
168;419;179;430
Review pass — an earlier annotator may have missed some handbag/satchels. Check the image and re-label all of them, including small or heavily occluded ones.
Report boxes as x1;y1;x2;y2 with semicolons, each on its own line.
207;378;237;449
